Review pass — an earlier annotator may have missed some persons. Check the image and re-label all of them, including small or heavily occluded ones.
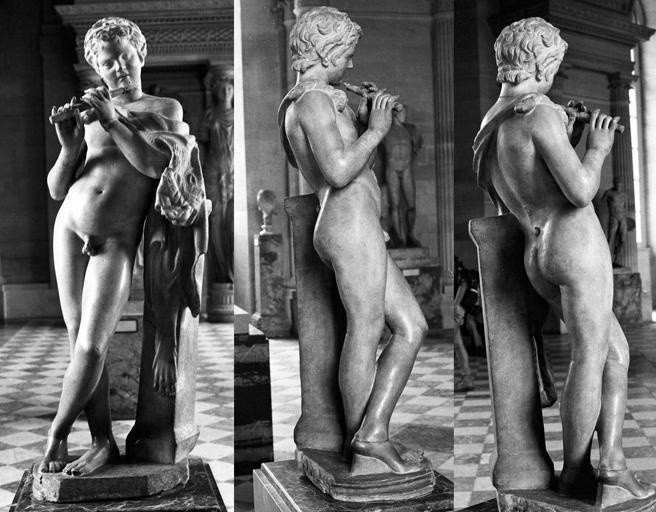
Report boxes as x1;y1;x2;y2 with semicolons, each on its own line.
195;63;235;286
377;101;426;250
275;5;434;480
255;188;282;226
470;13;655;508
599;175;631;269
36;12;189;481
